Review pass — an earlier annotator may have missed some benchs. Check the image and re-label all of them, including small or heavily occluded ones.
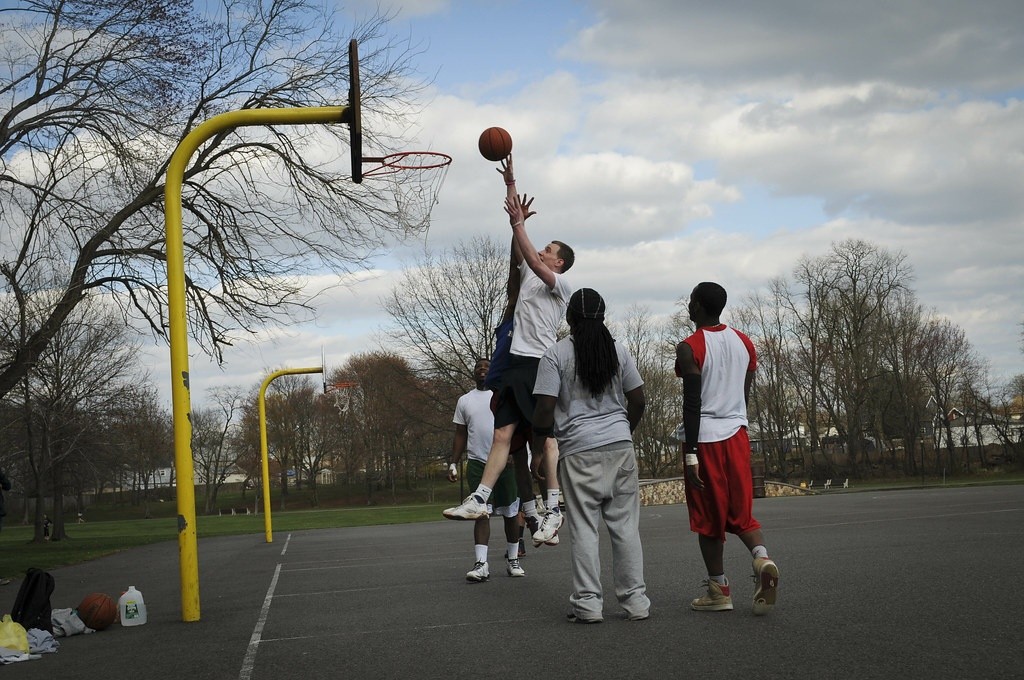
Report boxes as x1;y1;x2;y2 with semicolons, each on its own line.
219;505;252;516
808;478;848;490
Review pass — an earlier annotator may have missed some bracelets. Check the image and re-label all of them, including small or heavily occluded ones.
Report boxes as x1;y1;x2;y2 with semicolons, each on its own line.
686;454;699;465
513;220;523;227
506;180;516;186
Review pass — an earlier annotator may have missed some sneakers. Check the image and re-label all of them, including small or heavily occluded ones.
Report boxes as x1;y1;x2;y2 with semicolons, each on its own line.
750;557;780;614
690;576;735;610
507;558;525;577
525;515;560;548
504;544;526;558
533;510;564;541
442;491;490;521
465;561;489;581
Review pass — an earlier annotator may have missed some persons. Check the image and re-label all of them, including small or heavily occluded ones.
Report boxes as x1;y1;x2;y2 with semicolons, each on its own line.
43;514;52;539
504;441;532;558
78;512;84;524
674;281;780;614
530;288;651;623
442;153;572;549
447;359;526;582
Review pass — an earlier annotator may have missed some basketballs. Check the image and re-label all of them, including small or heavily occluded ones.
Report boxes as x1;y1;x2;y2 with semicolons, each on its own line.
479;127;512;162
79;593;117;630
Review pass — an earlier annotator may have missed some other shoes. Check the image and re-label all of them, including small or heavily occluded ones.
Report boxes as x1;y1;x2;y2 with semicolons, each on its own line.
566;613;603;623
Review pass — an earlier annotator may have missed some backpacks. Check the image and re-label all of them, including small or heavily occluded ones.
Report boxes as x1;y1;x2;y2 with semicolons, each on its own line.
12;567;55;634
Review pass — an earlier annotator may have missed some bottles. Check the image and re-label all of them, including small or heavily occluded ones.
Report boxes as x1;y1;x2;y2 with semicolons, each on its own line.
119;585;147;627
